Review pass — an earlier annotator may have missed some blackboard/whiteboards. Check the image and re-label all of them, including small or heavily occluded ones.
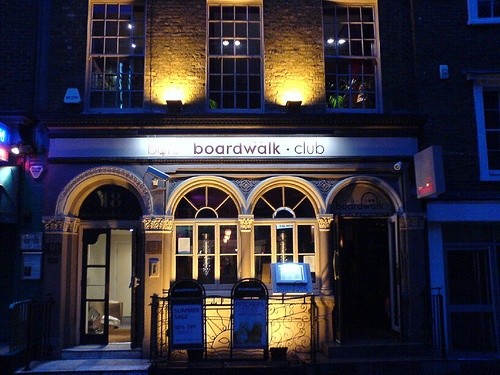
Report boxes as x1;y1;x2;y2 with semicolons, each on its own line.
230;277;269;349
168;279;206;350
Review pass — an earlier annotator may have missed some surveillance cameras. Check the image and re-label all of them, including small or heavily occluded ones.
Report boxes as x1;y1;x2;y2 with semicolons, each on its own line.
147;166;171;182
393;161;402;171
